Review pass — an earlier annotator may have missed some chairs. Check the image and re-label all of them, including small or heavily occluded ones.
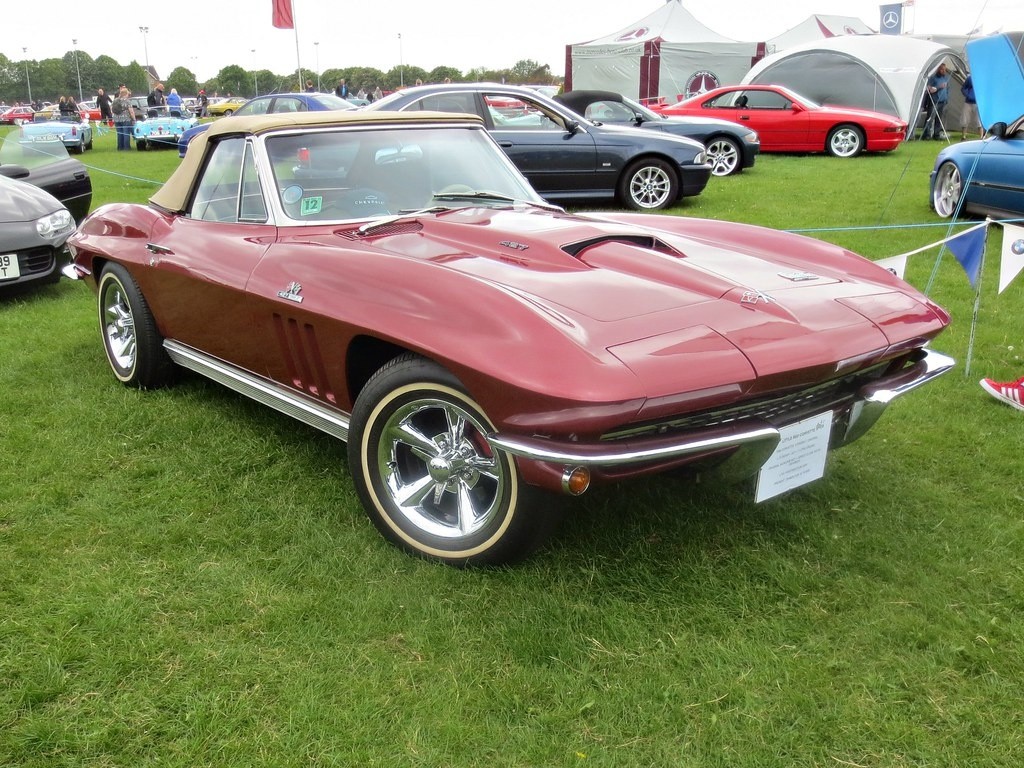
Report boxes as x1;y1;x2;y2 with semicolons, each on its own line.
367;146;431;211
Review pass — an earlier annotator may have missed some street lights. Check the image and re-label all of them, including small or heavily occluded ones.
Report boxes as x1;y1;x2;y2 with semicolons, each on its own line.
140;26;152;94
313;41;320;93
250;50;259;97
399;33;403;87
23;47;33;103
71;38;84;101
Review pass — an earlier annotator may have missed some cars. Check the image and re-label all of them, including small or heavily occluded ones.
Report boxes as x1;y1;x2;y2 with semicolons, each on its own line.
483;85;561;119
178;92;364;159
277;90;395;109
207;96;255;117
0;125;93;288
927;31;1024;227
0;95;115;128
128;95;225;127
292;82;714;213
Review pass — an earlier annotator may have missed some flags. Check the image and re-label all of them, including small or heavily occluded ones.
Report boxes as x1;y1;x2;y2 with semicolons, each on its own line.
272;0;293;30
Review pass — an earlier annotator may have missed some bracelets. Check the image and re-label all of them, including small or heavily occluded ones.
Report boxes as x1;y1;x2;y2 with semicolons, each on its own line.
132;119;136;121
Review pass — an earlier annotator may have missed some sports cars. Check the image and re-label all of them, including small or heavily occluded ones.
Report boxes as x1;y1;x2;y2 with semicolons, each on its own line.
131;105;200;151
18;109;93;155
494;90;762;178
644;83;909;159
64;110;962;572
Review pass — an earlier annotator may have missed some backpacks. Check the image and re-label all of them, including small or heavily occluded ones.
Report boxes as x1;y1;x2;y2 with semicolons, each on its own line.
111;97;130;114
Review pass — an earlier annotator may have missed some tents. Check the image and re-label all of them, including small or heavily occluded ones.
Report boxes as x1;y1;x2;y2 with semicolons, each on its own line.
564;0;1024;137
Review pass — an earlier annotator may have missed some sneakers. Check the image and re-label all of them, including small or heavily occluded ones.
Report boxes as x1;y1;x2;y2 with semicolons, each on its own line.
979;376;1024;411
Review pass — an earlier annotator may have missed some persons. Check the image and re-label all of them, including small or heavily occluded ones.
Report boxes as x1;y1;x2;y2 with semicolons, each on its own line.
332;78;348;100
199;91;209;119
213;92;230;98
306;80;314;93
15;102;24;107
357;87;382;103
97;89;113;118
59;96;79;116
1;102;5;106
416;78;422;87
444;78;450;84
147;83;182;117
921;63;949;140
960;75;986;141
111;84;136;150
31;99;44;111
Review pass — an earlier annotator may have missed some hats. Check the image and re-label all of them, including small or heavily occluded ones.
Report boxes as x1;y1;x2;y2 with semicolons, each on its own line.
345;187;387;218
